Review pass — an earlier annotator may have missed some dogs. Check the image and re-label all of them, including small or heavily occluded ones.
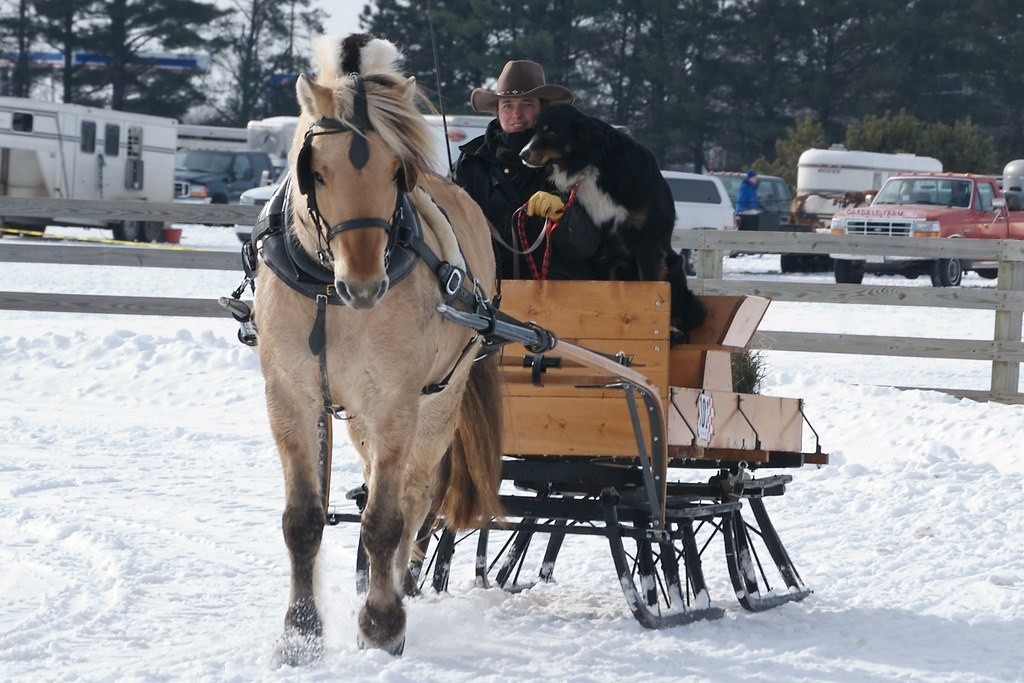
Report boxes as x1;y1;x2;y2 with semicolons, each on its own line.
521;100;711;332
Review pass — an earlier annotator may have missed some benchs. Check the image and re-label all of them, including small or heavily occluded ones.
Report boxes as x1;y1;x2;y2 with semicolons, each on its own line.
670;294;772;393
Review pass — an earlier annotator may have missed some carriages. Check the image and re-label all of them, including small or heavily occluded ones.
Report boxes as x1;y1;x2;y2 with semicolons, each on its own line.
213;27;832;668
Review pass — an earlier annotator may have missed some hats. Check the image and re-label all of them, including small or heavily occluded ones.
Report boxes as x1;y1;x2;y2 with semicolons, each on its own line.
470;60;575;113
747;171;756;178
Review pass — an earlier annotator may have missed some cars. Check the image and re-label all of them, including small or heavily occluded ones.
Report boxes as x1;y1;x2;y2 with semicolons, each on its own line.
699;170;793;228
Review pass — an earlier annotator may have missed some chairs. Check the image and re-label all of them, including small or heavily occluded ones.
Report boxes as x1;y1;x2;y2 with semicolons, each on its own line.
909;193;931;202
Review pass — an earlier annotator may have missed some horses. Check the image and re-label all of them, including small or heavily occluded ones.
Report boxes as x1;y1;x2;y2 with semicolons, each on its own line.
245;32;499;668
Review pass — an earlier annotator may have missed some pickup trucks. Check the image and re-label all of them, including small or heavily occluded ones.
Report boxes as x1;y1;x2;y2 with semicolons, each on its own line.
832;170;1024;289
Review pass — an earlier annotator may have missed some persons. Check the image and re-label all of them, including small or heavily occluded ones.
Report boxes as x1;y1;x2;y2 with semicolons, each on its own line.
443;59;594;280
947;181;969;206
736;170;764;230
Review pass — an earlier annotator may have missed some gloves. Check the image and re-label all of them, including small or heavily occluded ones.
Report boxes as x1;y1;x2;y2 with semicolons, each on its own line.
527;191;564;220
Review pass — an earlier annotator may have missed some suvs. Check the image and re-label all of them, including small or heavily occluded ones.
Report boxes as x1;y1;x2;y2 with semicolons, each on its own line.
172;148;274;229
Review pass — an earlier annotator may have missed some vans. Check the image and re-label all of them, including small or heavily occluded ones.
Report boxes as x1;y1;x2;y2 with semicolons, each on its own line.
653;168;738;276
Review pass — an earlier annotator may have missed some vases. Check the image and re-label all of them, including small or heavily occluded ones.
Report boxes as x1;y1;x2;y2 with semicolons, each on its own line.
161;229;181;243
